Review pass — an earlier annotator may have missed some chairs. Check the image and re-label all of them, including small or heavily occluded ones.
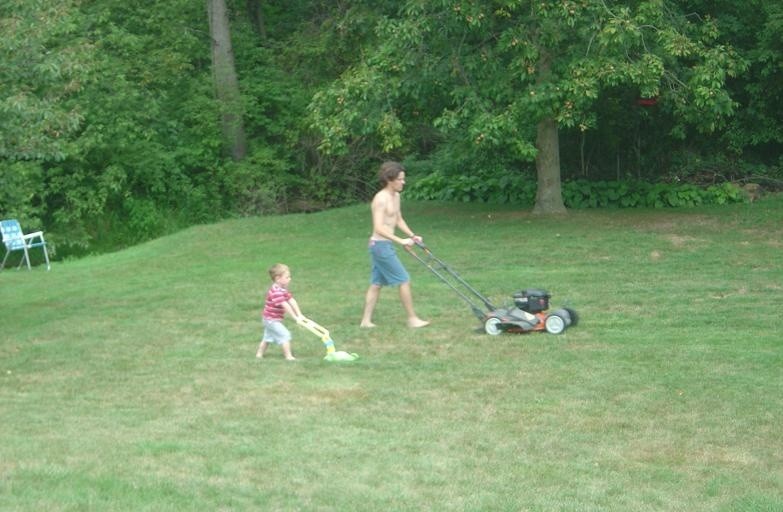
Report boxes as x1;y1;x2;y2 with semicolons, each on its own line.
0;219;50;271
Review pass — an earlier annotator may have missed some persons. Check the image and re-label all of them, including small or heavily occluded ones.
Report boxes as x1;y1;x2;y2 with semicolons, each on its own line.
357;160;433;329
254;261;306;361
